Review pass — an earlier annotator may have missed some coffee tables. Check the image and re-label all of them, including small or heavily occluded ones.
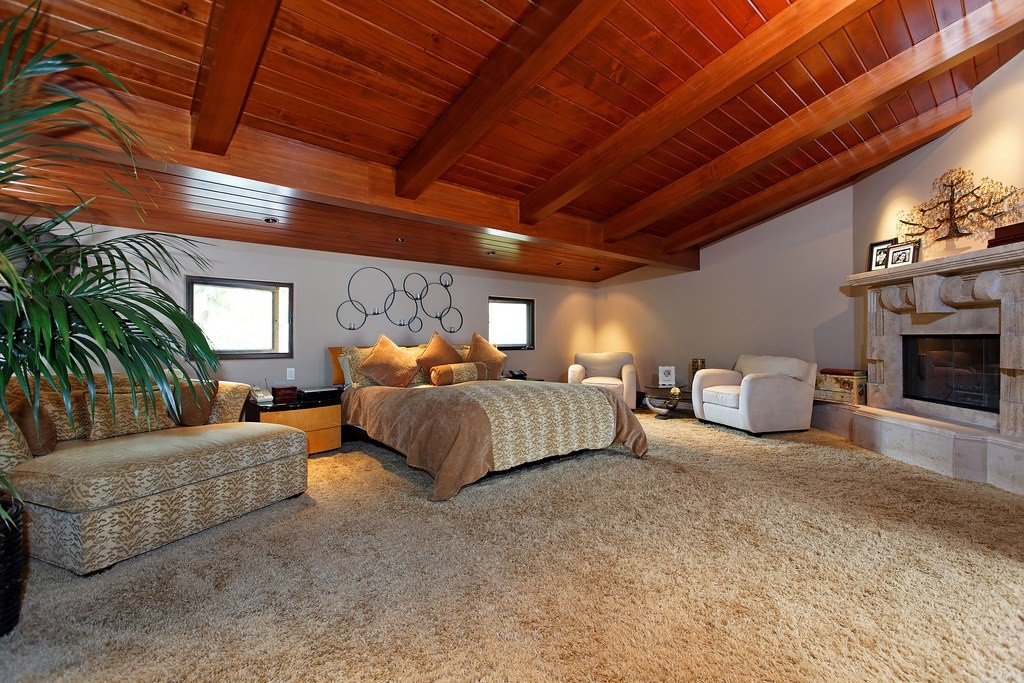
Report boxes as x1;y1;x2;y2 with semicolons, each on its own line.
644;385;692;419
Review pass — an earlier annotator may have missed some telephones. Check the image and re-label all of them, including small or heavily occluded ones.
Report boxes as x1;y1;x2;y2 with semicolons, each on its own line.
509;369;527;377
250;384;274;403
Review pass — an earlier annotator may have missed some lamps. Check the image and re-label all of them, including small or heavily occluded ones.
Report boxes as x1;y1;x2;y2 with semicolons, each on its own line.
692;359;706;385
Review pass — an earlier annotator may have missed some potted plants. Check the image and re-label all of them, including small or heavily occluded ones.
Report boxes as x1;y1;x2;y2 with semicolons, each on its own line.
0;1;222;637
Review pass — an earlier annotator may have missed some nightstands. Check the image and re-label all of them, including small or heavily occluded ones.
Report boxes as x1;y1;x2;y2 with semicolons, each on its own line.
506;376;544;381
245;397;342;455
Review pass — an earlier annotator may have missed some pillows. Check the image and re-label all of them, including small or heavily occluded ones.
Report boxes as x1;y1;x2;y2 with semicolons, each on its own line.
467;331;508;380
337;354;353;390
342;345;428;386
82;388;177;442
168;378;218;426
357;335;421;388
4;392;57;456
416;331;463;377
419;343;498;363
426;363;489;386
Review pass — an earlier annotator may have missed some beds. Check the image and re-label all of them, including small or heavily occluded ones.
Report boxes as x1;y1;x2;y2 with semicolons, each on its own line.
328;346;650;502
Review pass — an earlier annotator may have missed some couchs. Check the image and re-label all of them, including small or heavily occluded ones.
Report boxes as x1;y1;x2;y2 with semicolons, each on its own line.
0;371;310;580
568;351;637;412
692;354;818;437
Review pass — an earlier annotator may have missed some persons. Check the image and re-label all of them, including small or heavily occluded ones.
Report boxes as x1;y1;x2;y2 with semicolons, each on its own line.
875;250;887;266
663;371;670;381
894;252;907;263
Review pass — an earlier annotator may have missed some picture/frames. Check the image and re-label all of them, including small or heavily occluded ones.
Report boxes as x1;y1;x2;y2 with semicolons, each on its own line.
869;238;899;271
885;238;921;269
658;365;676;386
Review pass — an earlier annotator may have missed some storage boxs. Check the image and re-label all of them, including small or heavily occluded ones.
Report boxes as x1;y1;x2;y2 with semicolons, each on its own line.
813;373;867;405
272;385;297;399
